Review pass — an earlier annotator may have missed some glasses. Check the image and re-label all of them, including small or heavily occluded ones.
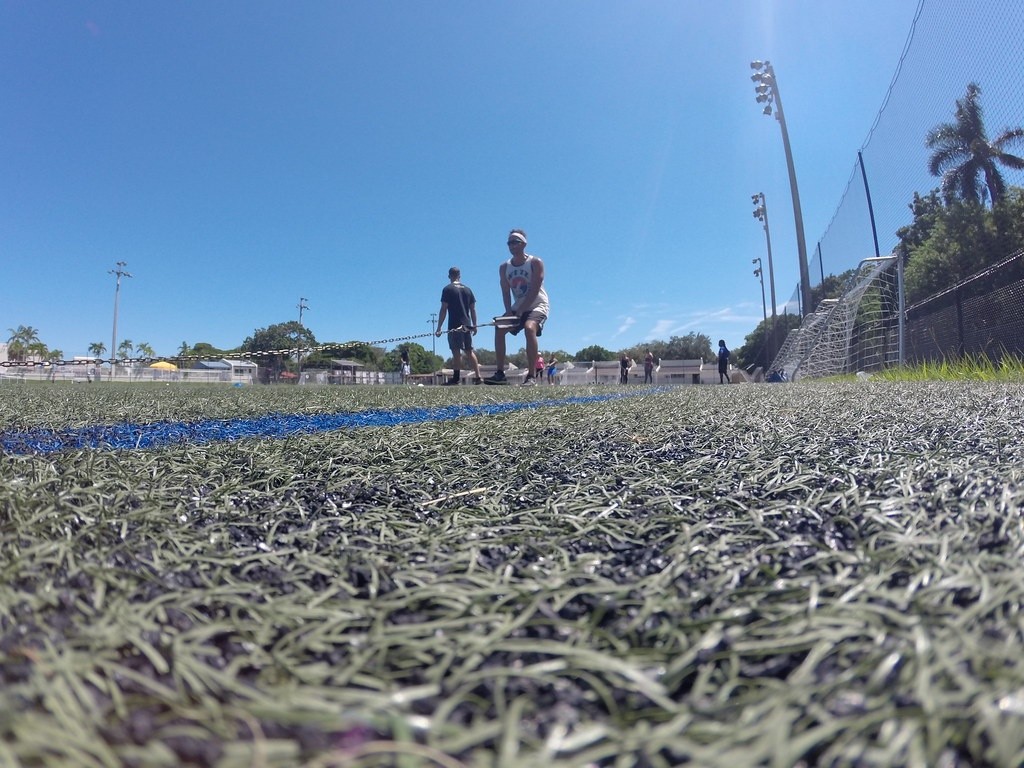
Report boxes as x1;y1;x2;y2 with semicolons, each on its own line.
507;239;522;245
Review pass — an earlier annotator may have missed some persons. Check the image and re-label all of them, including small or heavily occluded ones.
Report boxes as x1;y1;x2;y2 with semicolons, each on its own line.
484;229;548;386
435;267;483;386
718;340;731;385
400;351;411;385
620;351;631;383
644;349;653;384
536;352;544;384
547;353;558;385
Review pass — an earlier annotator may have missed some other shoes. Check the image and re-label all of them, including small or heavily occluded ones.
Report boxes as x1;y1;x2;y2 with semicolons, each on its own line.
474;377;483;385
522;375;536;386
443;378;461;386
484;373;507;385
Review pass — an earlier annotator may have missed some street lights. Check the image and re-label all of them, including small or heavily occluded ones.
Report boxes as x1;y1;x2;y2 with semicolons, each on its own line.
750;60;813;319
751;256;767;323
297;297;310;325
751;191;778;318
425;313;439;353
107;259;134;360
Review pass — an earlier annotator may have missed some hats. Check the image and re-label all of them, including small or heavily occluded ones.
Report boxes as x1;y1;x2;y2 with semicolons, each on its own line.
538;352;541;355
